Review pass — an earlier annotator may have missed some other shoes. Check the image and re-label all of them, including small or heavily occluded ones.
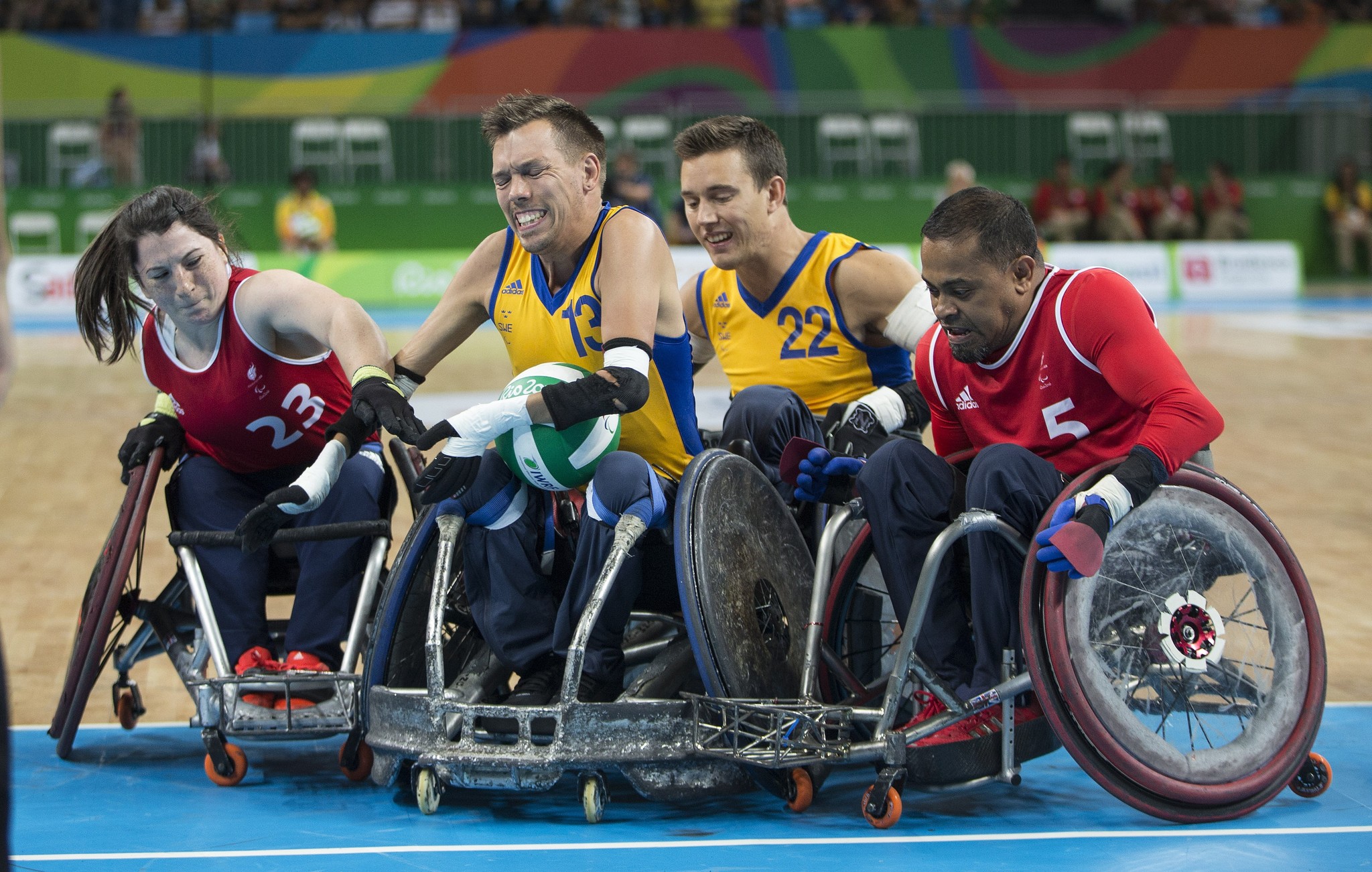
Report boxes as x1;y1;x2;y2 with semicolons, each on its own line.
273;651;332;712
482;673;563;738
235;645;282;709
539;670;625;737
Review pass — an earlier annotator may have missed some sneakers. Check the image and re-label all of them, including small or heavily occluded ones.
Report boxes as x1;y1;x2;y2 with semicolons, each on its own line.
895;689;948;731
906;704;1039;747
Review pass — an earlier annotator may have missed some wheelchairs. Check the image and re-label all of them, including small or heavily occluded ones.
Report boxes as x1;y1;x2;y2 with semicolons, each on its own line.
41;400;1334;833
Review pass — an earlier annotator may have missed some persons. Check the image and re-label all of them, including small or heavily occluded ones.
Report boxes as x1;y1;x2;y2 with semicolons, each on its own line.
72;186;425;720
772;186;1222;758
936;155;976;202
1320;159;1372;283
338;98;706;737
608;137;702;242
188;121;229;186
269;168;336;287
94;87;147;190
670;115;936;629
6;0;1372;47
1028;161;1250;249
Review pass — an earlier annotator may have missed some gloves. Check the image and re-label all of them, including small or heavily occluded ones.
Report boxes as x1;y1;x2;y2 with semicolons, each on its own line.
1036;494;1114;579
351;365;427;447
411;409;493;505
794;447;868;507
824;401;883;457
118;410;187;486
235;467;331;556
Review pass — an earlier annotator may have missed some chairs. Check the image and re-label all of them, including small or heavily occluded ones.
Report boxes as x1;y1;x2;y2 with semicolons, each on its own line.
46;121;102;190
591;115;677;181
289;113;395;185
819;111;923;179
1064;107;1174;174
